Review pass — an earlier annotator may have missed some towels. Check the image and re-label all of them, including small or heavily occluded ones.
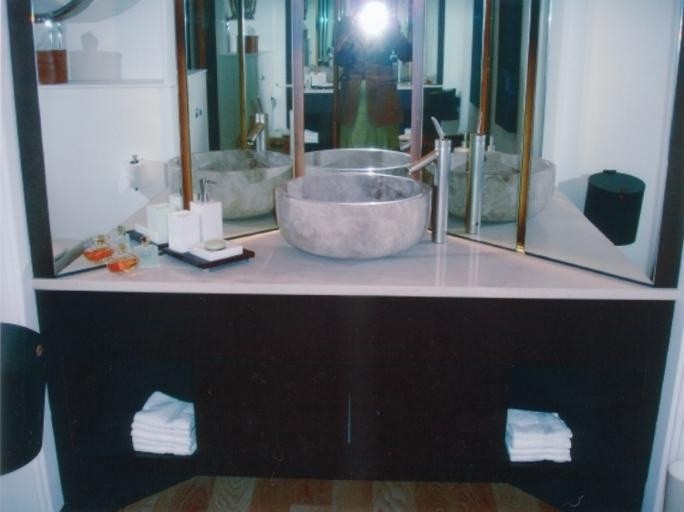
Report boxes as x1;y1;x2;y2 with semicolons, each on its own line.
505;408;573;464
130;392;198;457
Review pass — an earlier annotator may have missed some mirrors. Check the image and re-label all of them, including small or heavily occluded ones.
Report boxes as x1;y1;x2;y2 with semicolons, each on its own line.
31;0;190;278
305;1;411;82
175;0;540;256
525;1;679;289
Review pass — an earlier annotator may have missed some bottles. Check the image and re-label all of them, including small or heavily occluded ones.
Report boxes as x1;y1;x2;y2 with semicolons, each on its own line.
243;19;259;53
34;14;70;86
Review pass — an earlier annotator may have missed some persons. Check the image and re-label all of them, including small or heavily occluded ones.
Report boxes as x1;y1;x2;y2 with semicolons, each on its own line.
336;0;412;150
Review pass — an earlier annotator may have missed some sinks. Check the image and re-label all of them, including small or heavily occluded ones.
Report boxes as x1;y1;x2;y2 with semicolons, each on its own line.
166;149;293;220
423;147;556;223
275;172;432;261
303;147;410;177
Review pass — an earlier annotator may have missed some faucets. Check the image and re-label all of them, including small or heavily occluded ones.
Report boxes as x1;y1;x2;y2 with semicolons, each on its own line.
408;117;452;243
465;132;486;234
246;111;267;152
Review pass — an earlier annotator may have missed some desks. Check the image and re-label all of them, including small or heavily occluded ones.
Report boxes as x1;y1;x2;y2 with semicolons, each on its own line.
302;85;460;146
